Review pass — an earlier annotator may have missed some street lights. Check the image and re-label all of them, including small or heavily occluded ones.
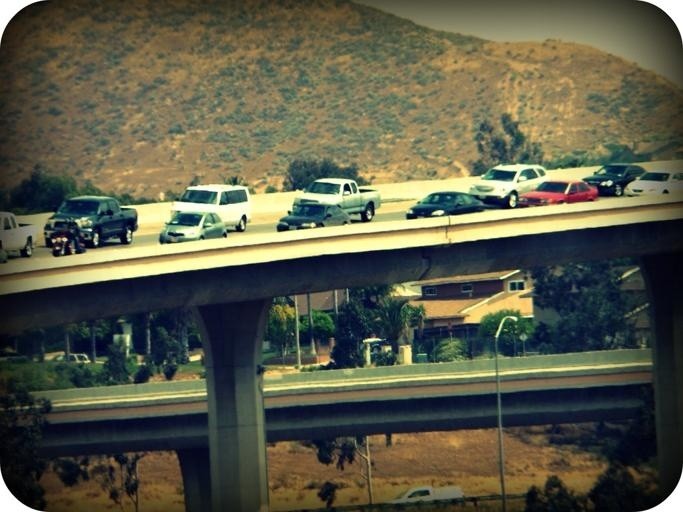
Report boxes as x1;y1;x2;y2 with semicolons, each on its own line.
494;315;518;511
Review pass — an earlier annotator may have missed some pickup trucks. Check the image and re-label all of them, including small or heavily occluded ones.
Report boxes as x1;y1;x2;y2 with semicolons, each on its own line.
0;211;37;264
293;177;381;221
44;195;138;248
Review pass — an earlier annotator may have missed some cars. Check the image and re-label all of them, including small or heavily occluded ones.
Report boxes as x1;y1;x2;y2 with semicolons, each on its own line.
160;211;226;243
406;163;682;219
277;197;351;231
54;353;91;363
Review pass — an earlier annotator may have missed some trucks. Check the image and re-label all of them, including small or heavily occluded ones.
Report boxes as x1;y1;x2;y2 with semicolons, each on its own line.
392;486;464;504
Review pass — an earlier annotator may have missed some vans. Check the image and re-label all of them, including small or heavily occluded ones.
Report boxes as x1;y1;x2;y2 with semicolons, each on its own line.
171;184;252;232
